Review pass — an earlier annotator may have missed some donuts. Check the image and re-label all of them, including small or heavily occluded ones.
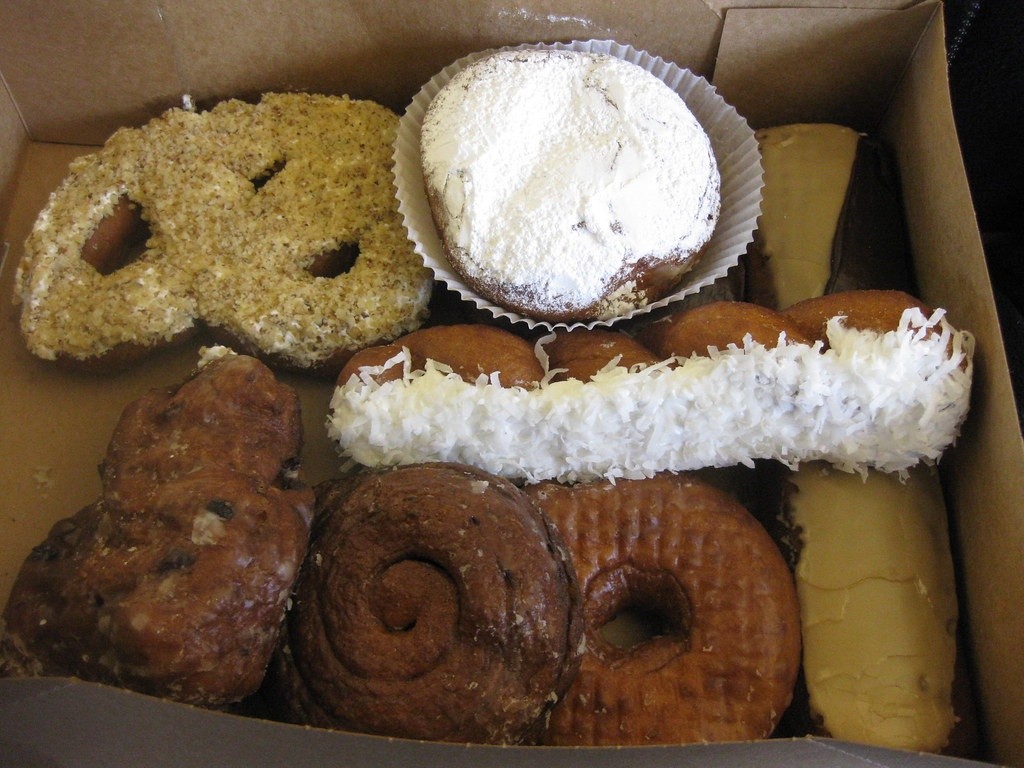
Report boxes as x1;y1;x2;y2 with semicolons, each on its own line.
525;477;800;746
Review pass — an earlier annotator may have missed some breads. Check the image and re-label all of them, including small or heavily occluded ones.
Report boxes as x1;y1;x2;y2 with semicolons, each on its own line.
0;350;573;746
328;283;975;481
741;120;976;764
12;88;433;365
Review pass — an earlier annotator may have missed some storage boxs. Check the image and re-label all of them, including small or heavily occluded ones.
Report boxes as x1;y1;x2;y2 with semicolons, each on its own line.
0;0;1023;768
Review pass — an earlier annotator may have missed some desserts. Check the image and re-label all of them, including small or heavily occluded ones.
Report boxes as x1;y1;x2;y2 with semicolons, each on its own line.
391;37;766;331
